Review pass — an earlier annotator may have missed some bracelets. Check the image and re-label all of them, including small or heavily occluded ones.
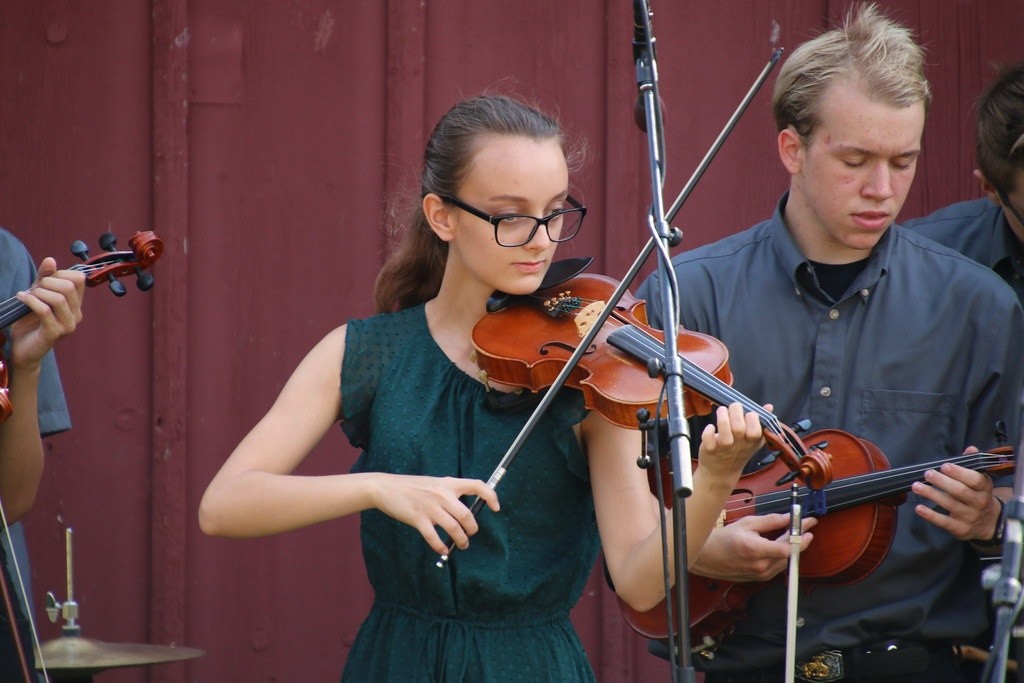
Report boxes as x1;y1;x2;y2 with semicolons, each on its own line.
970;495;1006;548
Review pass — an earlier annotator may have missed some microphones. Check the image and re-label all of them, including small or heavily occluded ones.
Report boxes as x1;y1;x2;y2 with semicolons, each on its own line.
631;0;668;131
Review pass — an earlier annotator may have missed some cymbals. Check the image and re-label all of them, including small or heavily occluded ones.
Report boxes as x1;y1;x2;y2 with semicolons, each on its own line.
33;635;208;678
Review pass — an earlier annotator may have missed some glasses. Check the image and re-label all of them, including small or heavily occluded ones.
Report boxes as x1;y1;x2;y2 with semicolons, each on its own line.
421;192;586;247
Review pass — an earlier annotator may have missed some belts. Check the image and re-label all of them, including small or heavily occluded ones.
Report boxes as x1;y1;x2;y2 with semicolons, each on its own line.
737;641;990;683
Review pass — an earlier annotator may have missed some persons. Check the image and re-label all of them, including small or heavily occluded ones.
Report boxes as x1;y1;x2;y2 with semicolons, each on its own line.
198;97;773;683
632;0;1024;683
0;225;86;683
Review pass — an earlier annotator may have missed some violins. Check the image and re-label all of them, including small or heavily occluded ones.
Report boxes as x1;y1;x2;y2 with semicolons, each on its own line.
469;273;835;492
0;229;166;422
617;428;1017;649
